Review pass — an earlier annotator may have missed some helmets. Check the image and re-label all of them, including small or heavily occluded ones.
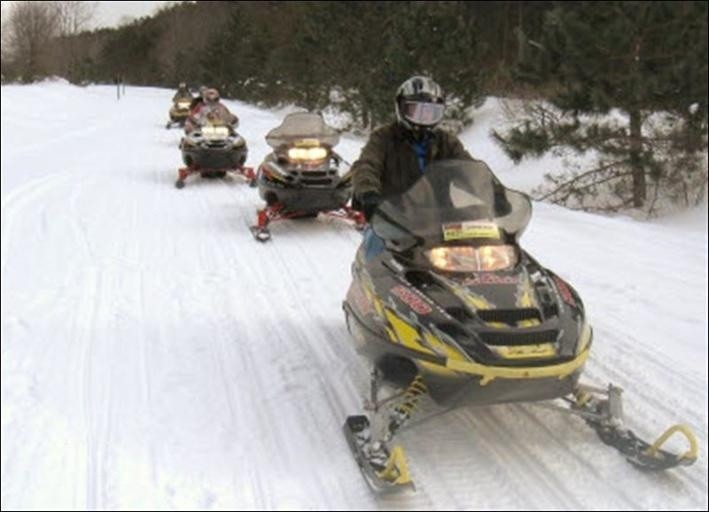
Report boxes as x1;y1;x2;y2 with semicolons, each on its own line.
199;87;220;104
179;83;187;89
394;76;445;133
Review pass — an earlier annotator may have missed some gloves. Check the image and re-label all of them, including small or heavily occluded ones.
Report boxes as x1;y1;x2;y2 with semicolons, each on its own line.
361;192;380;220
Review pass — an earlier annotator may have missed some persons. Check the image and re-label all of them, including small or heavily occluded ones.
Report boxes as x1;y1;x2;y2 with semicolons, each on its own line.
346;74;509;230
171;82;239;134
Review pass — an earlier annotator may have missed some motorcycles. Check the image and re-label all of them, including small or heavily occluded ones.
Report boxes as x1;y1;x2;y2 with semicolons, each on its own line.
342;159;700;498
249;111;369;242
173;101;258;191
165;97;204;131
